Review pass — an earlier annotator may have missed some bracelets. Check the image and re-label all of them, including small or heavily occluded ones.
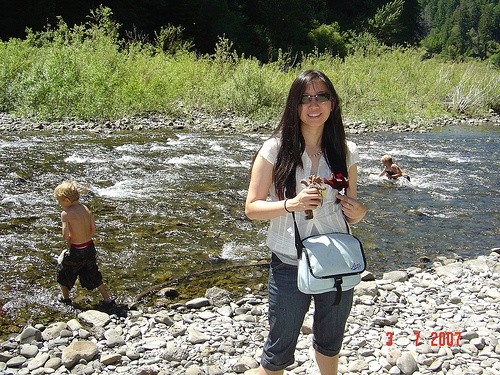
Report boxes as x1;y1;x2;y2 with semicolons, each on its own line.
283;198;292;214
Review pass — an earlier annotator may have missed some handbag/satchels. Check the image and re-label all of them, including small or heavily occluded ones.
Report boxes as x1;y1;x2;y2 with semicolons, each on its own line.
297;232;366;295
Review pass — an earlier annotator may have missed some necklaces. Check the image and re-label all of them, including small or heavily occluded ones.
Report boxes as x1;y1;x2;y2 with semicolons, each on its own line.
306;146;323;158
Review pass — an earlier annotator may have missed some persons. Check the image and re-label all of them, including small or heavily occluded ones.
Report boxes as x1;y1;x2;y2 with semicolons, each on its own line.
244;69;367;375
378;154;410;183
53;181;116;307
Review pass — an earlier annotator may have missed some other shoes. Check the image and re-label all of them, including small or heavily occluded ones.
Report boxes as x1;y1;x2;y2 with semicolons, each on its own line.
57;296;72;305
102;299;115;306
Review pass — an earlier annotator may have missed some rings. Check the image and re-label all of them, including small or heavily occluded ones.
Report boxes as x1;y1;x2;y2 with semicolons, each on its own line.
346;202;350;209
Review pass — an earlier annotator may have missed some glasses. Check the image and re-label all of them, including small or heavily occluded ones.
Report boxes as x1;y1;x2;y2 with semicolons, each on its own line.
299;93;330;104
